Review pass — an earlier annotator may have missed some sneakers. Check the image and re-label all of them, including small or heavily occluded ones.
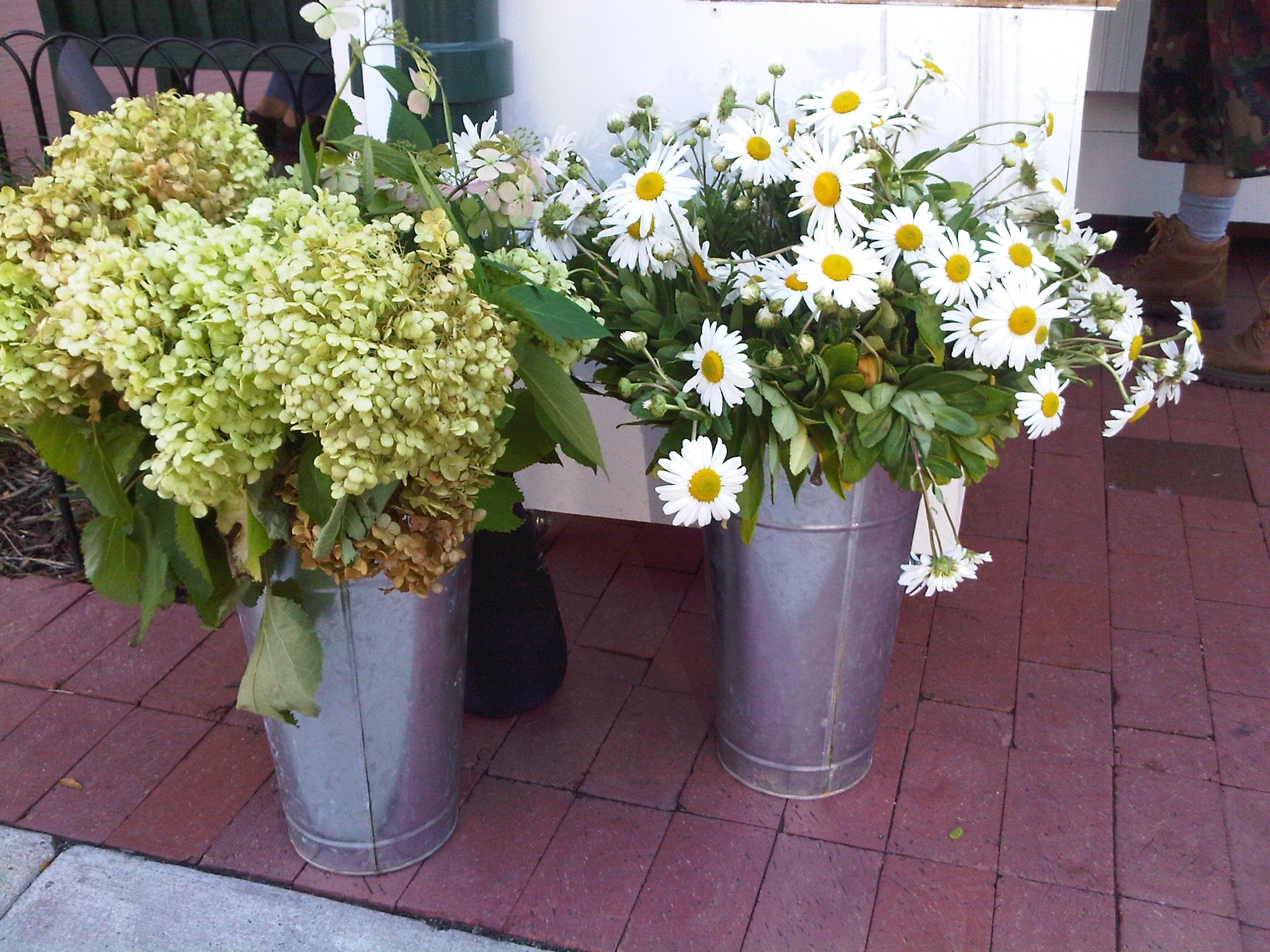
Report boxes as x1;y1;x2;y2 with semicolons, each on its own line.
1191;274;1270;390
1110;214;1232;329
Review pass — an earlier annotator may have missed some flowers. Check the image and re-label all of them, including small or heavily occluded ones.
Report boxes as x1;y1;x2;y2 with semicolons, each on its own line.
451;42;1208;603
0;0;606;730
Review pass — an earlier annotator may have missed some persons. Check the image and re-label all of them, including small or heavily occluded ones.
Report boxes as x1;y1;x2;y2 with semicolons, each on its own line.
245;72;337;154
1108;1;1269;392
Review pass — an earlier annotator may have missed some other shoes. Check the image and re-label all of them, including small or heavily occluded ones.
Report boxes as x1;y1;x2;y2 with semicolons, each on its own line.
246;104;328;171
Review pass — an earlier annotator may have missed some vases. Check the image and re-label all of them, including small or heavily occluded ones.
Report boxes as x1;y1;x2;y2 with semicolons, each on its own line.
445;464;565;725
